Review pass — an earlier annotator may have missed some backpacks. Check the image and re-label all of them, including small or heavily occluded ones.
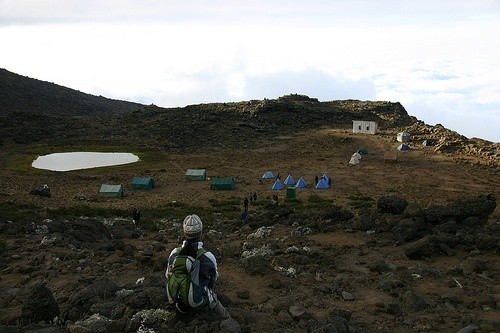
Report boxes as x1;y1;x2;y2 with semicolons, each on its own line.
166;247;207;309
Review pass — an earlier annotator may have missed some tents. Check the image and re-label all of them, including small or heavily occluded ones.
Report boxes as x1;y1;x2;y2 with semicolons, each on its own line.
422;139;431;146
185;169;206;180
295;177;307;188
315;173;329;189
396;144;407;151
98;184;123;198
349;148;369;165
131;177;155;190
397;132;410;142
262;171;275;178
209;177;236;190
271;179;284;190
283;175;295;184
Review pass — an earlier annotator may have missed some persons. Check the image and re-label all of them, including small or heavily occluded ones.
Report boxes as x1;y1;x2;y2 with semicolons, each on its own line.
132;207;141;227
244;192;257;211
163;214;220;313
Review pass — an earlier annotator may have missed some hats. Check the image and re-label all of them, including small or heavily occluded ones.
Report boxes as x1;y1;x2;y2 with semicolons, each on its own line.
182;214;202;234
